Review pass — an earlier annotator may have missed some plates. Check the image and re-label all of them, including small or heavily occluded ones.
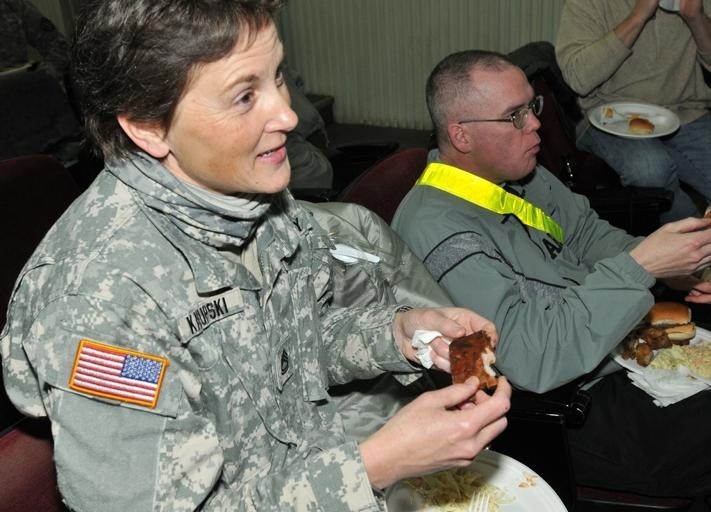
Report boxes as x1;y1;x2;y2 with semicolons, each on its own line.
387;449;569;512
587;101;681;139
610;324;711;381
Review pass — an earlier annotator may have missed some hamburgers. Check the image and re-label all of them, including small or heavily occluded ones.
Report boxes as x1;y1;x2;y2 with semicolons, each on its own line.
646;302;695;340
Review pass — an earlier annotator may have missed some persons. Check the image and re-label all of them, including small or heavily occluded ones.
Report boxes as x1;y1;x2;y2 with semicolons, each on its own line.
0;2;514;510
1;2;106;299
387;48;711;510
557;2;711;246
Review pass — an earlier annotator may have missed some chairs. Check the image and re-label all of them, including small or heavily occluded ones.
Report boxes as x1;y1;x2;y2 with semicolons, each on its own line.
0;154;78;331
497;37;678;229
339;144;702;512
0;410;82;512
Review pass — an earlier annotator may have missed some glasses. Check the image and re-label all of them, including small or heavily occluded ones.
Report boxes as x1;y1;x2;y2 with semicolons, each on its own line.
458;93;543;129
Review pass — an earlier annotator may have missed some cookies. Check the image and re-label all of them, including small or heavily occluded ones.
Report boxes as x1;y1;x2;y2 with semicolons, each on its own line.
448;329;496;393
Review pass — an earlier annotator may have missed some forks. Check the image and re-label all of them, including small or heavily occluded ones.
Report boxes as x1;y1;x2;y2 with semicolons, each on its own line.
468;489;491;512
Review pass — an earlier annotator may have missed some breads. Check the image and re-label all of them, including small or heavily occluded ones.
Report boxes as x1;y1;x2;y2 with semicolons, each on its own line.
629;118;656;135
